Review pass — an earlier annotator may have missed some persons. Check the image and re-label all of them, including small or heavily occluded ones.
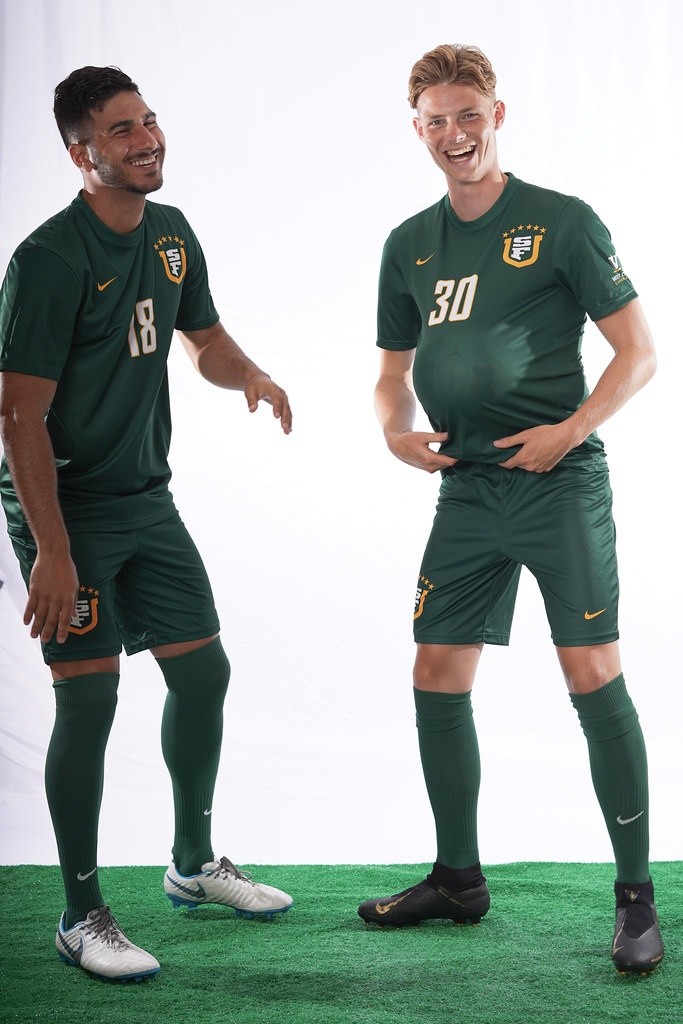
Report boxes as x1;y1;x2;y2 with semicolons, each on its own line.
358;38;666;976
0;66;297;986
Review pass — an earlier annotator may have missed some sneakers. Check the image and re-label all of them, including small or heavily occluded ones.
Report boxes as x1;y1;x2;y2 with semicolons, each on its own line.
162;856;293;920
358;876;491;928
611;877;664;976
54;906;160;984
